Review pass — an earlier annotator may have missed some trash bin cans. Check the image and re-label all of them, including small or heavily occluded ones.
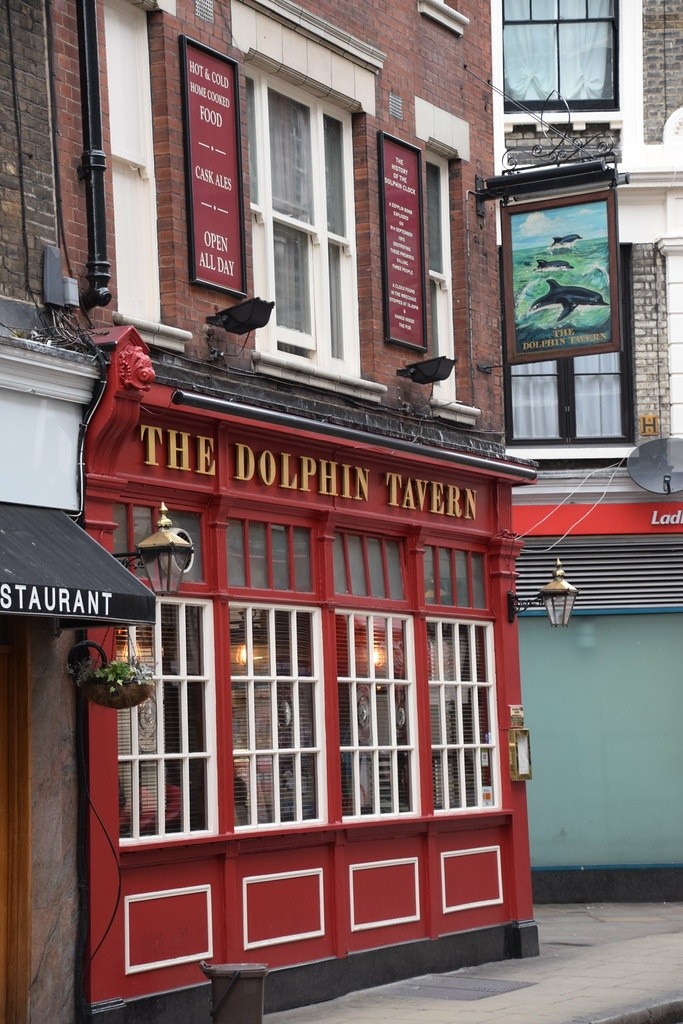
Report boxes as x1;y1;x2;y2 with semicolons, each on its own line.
199;960;269;1024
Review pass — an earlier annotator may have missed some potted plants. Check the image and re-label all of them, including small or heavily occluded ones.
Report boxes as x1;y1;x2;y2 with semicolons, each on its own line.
79;627;155;707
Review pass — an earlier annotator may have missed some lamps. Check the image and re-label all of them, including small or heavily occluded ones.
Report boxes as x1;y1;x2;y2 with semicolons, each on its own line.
373;644;385;668
122;639;142;662
204;297;277;358
506;558;579;628
236;643;247;666
395;354;457;412
108;501;193;597
